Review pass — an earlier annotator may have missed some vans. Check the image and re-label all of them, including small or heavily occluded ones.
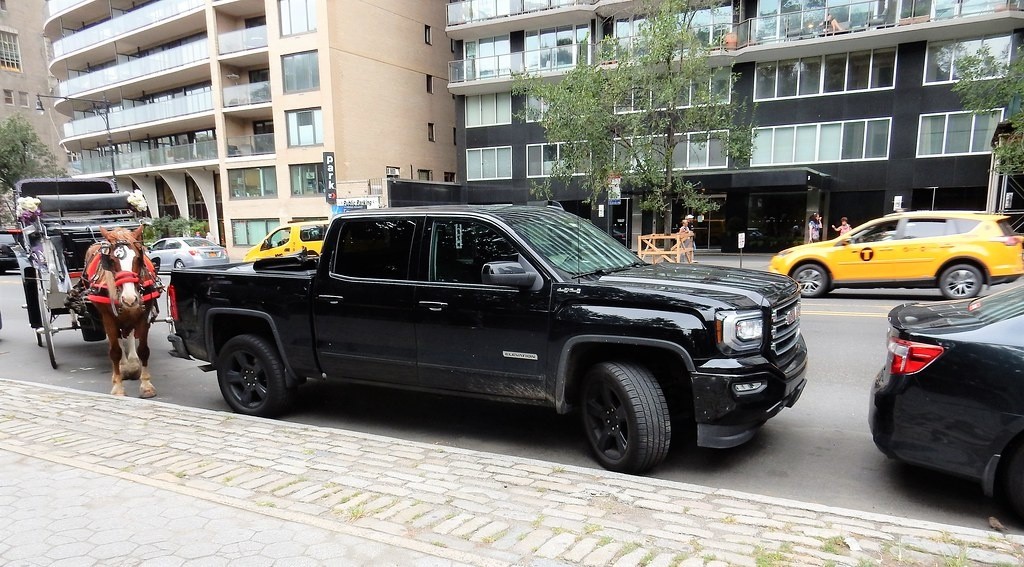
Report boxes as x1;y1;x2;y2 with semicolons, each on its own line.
241;220;333;263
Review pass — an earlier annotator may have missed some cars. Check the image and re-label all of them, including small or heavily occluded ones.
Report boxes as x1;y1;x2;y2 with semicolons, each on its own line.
0;230;24;275
144;236;230;274
866;278;1024;523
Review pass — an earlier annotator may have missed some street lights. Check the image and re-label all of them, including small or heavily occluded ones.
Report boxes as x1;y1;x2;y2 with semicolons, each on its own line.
34;92;119;193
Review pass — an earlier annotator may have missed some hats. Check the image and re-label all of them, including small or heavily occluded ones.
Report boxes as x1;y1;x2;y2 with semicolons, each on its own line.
688;215;694;219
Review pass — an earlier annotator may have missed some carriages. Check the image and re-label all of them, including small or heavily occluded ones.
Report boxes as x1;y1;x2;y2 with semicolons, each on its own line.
9;177;165;399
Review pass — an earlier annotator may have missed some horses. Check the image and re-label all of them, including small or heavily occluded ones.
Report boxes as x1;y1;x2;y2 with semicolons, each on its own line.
82;225;159;398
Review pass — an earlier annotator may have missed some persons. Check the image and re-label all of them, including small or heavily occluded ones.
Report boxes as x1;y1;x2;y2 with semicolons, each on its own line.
808;213;852;246
673;214;698;264
194;230;216;245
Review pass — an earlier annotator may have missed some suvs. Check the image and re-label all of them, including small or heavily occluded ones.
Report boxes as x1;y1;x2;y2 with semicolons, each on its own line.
769;208;1024;300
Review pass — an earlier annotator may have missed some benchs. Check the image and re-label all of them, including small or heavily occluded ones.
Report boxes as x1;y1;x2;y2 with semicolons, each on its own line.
34;194;146;269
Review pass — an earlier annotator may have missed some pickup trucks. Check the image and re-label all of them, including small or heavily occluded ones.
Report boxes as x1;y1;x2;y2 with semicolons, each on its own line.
164;204;810;477
